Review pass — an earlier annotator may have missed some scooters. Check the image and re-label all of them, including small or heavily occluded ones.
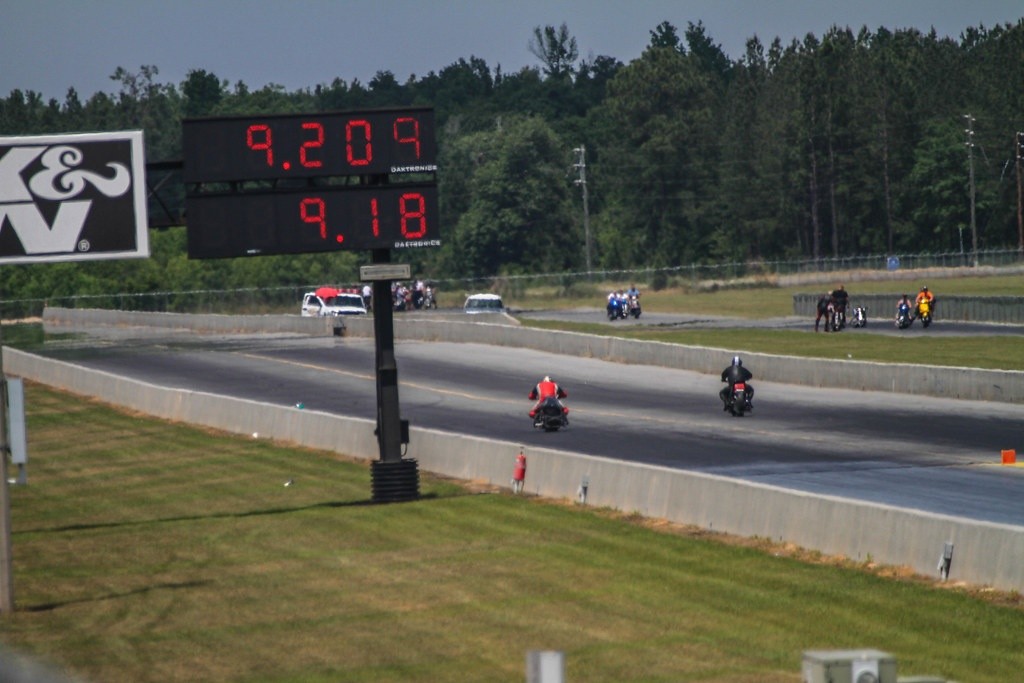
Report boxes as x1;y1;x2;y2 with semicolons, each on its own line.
606;295;642;320
851;305;869;328
916;297;934;329
898;303;911;329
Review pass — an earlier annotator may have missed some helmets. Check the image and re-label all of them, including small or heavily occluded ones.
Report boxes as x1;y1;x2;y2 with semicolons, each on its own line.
732;355;742;366
902;293;908;297
543;375;552;381
922;286;928;291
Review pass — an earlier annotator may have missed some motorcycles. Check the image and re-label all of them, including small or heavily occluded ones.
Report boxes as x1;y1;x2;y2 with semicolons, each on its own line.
532;396;570;433
721;380;752;417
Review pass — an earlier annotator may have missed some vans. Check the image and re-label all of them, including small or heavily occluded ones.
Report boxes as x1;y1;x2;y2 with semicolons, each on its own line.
301;291;371;316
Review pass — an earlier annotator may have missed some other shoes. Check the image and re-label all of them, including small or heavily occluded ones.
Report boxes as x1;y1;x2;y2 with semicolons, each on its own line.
723;402;730;411
746;399;753;409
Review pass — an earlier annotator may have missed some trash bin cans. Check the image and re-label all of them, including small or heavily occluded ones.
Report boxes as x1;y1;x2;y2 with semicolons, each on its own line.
887;257;899;271
334;327;346;336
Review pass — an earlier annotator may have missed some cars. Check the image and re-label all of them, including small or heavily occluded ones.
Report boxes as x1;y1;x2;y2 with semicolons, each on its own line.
461;293;507;315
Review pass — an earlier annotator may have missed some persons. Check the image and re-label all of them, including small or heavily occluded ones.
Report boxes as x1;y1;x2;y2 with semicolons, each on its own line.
719;355;754;409
815;290;833;332
911;286;936;323
831;285;849;327
895;293;912;324
362;285;372;312
392;278;425;308
625;284;640;298
608;291;619;302
528;376;569;417
618;289;628;299
